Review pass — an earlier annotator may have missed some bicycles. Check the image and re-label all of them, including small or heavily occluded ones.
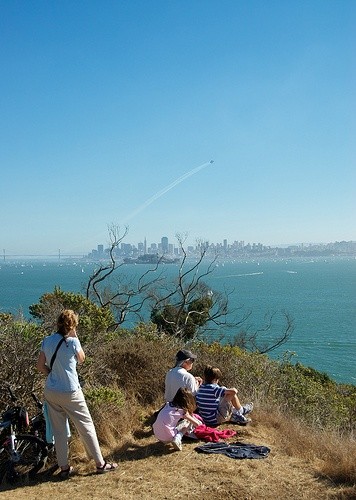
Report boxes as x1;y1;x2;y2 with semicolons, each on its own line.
0;384;55;487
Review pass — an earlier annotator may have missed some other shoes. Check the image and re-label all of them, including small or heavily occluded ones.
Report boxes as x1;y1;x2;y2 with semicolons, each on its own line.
172;434;182;451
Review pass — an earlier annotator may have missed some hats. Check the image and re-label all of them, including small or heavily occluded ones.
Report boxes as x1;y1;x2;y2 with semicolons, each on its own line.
176;349;197;361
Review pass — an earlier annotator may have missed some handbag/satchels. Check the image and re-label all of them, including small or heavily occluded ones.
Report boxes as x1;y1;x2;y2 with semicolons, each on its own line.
44;402;71;443
143;403;166;426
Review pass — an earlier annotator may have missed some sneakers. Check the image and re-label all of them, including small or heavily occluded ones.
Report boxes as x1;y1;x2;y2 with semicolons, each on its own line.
242;402;253;415
230;414;252;424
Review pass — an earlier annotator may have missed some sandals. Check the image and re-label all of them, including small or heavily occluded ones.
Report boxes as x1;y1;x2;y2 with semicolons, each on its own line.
96;460;118;474
60;465;75;478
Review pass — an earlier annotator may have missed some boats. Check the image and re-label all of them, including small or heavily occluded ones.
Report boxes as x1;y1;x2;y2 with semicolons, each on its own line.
124;253;183;265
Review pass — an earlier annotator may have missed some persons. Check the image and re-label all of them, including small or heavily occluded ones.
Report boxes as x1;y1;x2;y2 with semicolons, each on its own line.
36;310;118;476
152;387;203;452
164;349;203;422
194;365;254;428
